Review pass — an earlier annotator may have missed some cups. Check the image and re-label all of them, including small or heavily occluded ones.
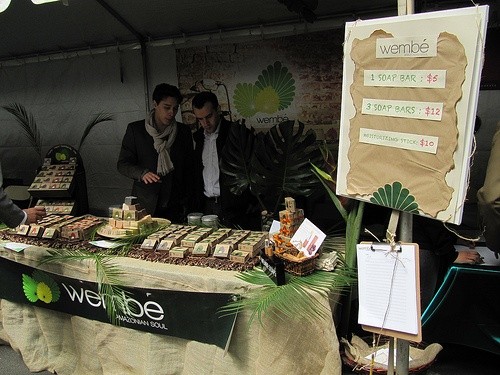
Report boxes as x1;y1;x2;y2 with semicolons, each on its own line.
202;214;218;229
186;212;203;228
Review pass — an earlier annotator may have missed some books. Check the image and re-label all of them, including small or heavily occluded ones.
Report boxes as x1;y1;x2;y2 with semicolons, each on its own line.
454;244;500;266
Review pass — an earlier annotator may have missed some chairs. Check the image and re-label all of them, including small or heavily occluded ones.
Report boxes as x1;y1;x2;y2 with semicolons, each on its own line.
4;185;33;209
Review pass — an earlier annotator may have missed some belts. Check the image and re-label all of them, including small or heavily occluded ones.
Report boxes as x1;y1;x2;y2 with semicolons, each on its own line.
205;197;220;203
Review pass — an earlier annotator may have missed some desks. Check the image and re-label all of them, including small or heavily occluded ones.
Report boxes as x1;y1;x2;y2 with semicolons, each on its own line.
0;219;353;375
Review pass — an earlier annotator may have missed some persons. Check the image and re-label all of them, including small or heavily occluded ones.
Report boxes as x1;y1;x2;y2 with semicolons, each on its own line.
116;83;195;225
412;114;482;315
191;92;274;231
475;121;500;252
0;187;47;228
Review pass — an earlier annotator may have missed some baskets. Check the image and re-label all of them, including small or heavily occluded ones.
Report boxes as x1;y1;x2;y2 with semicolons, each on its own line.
276;248;319;277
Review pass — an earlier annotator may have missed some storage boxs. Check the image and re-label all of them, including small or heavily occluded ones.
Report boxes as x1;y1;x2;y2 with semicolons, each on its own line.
16;197;305;264
